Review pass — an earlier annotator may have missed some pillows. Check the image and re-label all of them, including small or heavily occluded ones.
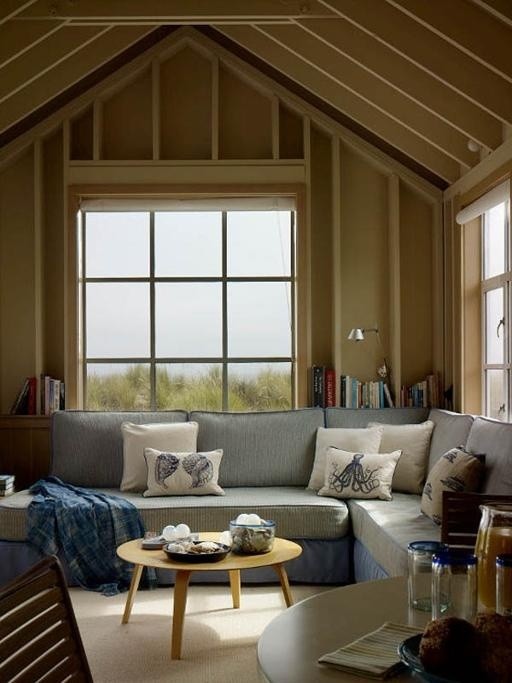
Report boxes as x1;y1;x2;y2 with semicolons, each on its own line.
306;424;383;494
423;442;486;525
114;419;199;492
315;444;402;500
360;416;435;498
142;446;225;499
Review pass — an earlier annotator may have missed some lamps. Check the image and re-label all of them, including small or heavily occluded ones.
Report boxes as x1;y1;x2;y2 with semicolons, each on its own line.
347;324;392;390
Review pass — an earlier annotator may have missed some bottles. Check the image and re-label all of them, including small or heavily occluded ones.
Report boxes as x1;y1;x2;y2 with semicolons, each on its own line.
473;502;511;612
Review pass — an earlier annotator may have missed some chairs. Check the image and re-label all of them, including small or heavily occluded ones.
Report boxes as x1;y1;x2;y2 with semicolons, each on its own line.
440;490;511;573
0;558;95;682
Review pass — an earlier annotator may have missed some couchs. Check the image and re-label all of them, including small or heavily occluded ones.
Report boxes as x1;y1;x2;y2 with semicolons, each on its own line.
1;404;511;591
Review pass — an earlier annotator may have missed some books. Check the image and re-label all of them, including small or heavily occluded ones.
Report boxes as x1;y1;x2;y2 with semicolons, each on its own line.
8;376;65;416
0;474;15;496
311;366;439;409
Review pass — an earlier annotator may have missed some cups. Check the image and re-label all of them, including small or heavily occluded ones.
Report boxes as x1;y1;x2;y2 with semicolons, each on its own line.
428;548;479;621
495;553;512;615
405;539;451;612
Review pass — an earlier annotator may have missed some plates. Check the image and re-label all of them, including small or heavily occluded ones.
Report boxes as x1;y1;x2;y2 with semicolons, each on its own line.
164;542;230;563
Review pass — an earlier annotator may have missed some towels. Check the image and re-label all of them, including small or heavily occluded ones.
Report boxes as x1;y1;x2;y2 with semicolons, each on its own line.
318;620;426;680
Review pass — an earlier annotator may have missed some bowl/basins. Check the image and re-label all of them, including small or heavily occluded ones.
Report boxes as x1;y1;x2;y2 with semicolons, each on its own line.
229;519;276;555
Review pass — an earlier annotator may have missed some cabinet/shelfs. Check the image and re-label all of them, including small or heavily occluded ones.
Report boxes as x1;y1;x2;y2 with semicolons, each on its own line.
1;412;53;492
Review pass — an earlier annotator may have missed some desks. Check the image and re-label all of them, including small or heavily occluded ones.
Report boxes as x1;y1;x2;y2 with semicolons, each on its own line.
258;574;510;682
